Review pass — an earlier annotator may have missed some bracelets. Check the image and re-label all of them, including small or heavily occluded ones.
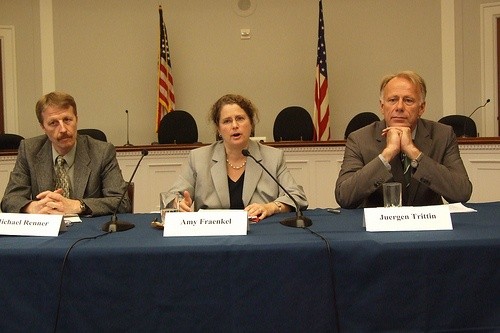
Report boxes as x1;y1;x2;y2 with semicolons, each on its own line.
78;199;87;214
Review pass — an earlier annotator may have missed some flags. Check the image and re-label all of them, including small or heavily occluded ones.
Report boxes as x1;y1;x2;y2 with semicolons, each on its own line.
156;7;176;133
313;0;331;142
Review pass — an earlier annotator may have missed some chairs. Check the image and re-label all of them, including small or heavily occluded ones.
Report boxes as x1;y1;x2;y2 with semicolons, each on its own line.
437;115;477;136
158;111;198;143
273;106;313;141
0;133;24;150
344;111;380;138
77;129;107;142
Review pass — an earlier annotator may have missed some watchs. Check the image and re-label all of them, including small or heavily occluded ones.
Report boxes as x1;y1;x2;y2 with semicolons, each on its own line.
411;152;424;168
273;201;282;212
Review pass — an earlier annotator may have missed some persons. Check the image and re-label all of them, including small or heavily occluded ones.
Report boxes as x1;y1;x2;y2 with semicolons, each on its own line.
335;71;473;209
0;91;132;218
166;94;310;220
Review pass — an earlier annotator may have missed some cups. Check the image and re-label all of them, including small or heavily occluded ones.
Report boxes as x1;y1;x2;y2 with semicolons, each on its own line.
159;192;180;226
382;183;402;207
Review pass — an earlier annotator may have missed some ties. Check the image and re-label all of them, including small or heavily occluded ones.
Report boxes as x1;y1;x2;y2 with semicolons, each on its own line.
55;155;71;200
402;152;412;187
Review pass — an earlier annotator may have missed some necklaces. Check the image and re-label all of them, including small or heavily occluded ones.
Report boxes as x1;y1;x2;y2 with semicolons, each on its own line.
227;157;248;169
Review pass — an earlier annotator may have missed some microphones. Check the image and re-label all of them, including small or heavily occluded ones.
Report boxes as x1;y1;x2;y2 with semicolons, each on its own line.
242;149;313;228
103;149;148;231
122;110;135;148
461;99;490;137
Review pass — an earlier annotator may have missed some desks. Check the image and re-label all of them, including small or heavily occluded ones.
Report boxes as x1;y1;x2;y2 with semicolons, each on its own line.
5;136;500;213
0;202;500;333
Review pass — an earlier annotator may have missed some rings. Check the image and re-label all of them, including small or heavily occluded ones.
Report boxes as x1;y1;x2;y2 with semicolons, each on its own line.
259;208;262;213
